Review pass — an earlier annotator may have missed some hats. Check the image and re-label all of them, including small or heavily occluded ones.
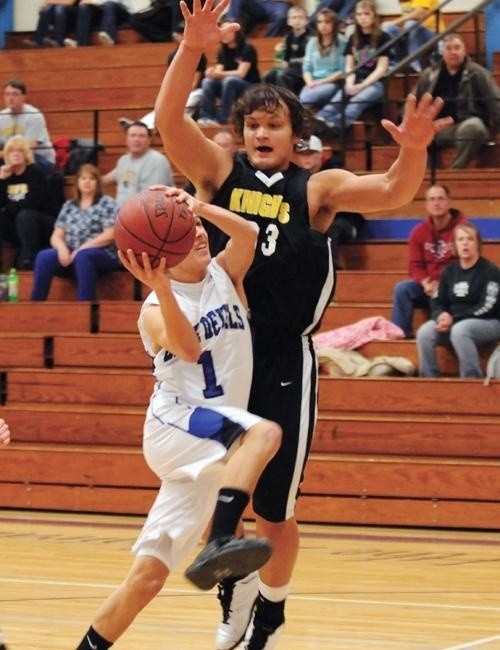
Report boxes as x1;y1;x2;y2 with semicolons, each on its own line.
297;135;322;154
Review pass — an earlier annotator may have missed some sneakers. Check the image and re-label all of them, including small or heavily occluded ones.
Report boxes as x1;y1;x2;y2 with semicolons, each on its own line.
23;38;78;48
118;118;133;128
186;537;268;590
216;574;285;650
100;31;112;46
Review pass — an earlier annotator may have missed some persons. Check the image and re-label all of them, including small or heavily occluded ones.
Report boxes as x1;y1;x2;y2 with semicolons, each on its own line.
155;0;455;650
170;0;500;170
295;135;364;270
74;186;284;650
391;184;500;378
21;0;193;47
0;80;173;299
0;419;11;449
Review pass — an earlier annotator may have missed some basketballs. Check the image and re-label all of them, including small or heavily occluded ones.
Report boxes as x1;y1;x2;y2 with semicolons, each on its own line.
113;187;197;269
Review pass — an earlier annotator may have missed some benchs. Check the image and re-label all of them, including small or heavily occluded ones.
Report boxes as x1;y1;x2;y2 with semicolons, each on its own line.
0;11;500;530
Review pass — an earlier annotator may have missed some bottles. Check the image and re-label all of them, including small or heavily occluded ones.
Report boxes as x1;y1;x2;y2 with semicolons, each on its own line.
8;267;19;300
